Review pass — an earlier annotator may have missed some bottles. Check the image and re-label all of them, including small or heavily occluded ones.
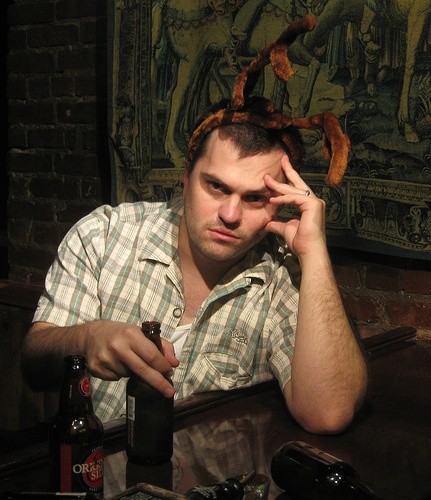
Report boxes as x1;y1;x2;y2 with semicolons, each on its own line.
48;354;106;500
126;321;172;467
268;440;385;500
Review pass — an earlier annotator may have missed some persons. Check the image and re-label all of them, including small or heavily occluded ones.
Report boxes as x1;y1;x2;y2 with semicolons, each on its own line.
23;96;366;436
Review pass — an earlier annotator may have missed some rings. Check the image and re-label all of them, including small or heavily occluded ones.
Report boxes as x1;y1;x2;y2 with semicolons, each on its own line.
304;189;312;197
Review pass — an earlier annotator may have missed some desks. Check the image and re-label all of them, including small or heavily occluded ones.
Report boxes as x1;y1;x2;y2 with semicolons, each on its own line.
0;326;431;500
